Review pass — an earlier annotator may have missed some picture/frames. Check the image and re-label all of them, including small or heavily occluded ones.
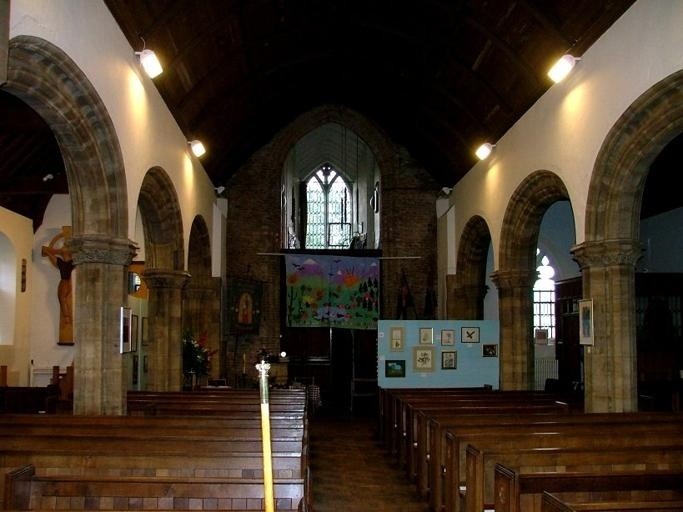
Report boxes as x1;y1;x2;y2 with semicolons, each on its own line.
383;326;457;378
576;296;594;347
118;305;149;355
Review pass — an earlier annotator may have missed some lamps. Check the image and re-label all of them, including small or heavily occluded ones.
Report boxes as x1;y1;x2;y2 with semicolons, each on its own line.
134;48;164;81
546;54;582;85
186;139;206;159
474;141;496;161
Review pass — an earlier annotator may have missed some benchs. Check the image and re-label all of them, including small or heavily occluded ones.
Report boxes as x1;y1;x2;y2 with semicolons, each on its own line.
392;388;682;512
0;384;312;512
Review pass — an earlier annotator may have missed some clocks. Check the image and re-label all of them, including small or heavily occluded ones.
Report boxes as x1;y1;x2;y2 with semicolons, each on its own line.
460;326;480;344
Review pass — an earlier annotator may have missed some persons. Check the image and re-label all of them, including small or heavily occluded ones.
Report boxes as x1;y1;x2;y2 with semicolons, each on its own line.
43;247;74;347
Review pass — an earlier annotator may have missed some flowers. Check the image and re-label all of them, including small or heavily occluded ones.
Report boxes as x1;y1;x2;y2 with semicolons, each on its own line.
181;327;217;376
